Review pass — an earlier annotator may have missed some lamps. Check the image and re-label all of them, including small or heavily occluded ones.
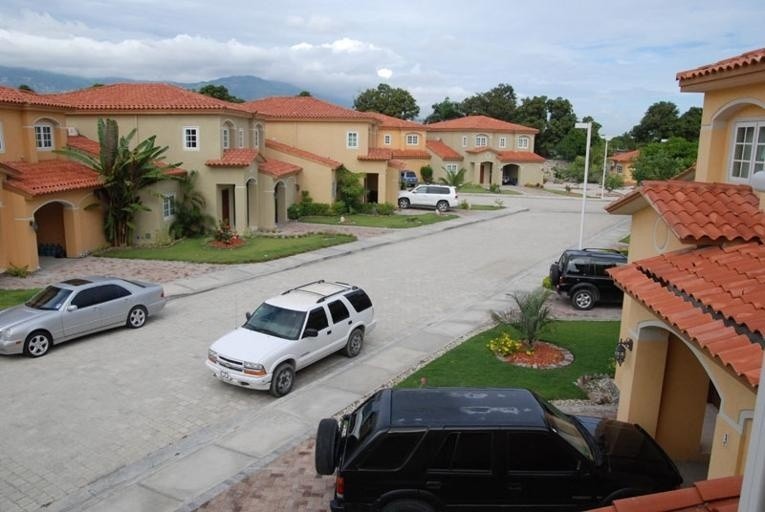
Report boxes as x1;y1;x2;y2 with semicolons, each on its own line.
616;337;633;366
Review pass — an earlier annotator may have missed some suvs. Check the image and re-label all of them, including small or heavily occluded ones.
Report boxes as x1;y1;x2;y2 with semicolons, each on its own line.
315;389;684;512
399;182;459;212
549;247;627;310
206;278;376;399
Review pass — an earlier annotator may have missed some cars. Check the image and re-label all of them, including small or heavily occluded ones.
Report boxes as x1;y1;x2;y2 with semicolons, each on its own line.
503;175;517;184
0;275;168;359
401;169;417;186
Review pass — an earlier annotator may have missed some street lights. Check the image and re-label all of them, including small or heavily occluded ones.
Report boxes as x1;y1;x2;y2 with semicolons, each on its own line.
572;121;594;251
599;133;612;198
